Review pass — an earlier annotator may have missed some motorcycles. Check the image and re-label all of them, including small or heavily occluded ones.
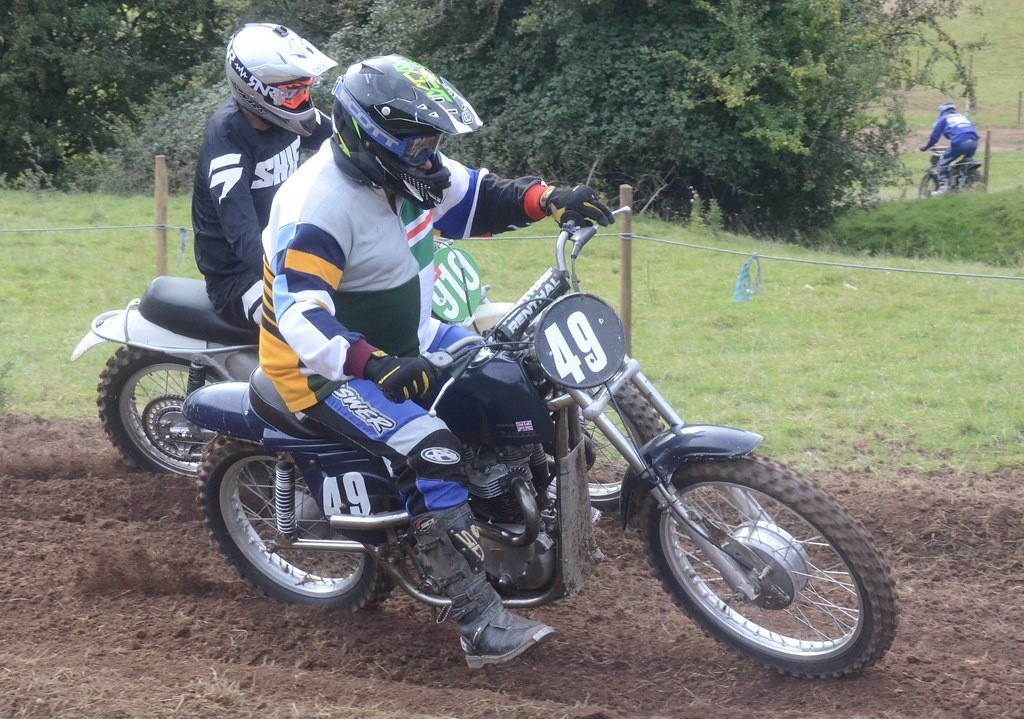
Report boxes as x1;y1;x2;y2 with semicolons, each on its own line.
67;232;666;512
916;144;986;199
181;204;901;680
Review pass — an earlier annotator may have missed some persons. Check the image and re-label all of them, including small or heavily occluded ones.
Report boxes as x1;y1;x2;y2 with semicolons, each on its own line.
192;22;336;332
918;102;981;196
263;54;616;668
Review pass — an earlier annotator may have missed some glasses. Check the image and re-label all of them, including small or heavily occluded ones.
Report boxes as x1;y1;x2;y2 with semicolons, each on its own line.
331;76;443;167
226;30;314;108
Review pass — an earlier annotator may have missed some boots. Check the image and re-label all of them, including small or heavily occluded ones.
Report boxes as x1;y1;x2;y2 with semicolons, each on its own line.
931;175;948;196
409;502;555;668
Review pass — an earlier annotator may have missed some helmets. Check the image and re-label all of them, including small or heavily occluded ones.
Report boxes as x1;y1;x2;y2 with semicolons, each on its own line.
330;54;482;210
938;102;956;115
226;23;339;137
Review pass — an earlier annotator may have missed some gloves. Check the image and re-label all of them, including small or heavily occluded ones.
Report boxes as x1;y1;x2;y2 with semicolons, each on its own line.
362;351;433;404
539;184;615;240
919;144;928;151
